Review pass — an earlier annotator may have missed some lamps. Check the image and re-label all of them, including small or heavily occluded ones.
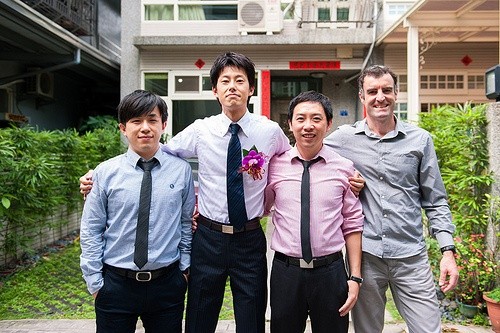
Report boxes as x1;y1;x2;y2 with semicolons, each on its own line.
310;72;328;78
485;65;500;102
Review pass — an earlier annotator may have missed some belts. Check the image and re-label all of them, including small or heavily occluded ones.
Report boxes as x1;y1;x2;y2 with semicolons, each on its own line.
103;260;180;281
274;251;343;269
198;215;260;234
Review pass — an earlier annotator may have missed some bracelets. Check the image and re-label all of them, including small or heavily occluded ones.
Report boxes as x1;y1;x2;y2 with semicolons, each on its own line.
349;276;363;283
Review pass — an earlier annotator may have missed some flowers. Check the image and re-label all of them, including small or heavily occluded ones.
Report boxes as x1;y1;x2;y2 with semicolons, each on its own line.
243;147;269;180
455;233;491;305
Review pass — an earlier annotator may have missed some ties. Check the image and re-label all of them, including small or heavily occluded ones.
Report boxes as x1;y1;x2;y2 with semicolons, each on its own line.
134;158;160;270
226;123;247;230
295;156;323;264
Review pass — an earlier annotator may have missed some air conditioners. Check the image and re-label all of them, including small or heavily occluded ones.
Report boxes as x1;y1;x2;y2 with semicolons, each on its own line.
27;67;54;97
238;0;284;31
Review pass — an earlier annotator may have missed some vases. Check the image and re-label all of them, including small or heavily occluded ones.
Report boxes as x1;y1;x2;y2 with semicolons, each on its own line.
456;297;478;317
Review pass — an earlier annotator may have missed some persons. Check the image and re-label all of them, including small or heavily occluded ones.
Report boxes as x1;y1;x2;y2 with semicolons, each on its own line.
323;66;459;333
79;90;195;333
192;91;364;333
79;52;363;333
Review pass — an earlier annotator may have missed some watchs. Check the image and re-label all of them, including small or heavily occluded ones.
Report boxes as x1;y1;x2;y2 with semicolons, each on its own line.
441;245;456;254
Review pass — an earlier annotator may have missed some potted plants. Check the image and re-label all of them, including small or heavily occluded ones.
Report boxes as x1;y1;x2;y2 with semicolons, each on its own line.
483;288;500;333
